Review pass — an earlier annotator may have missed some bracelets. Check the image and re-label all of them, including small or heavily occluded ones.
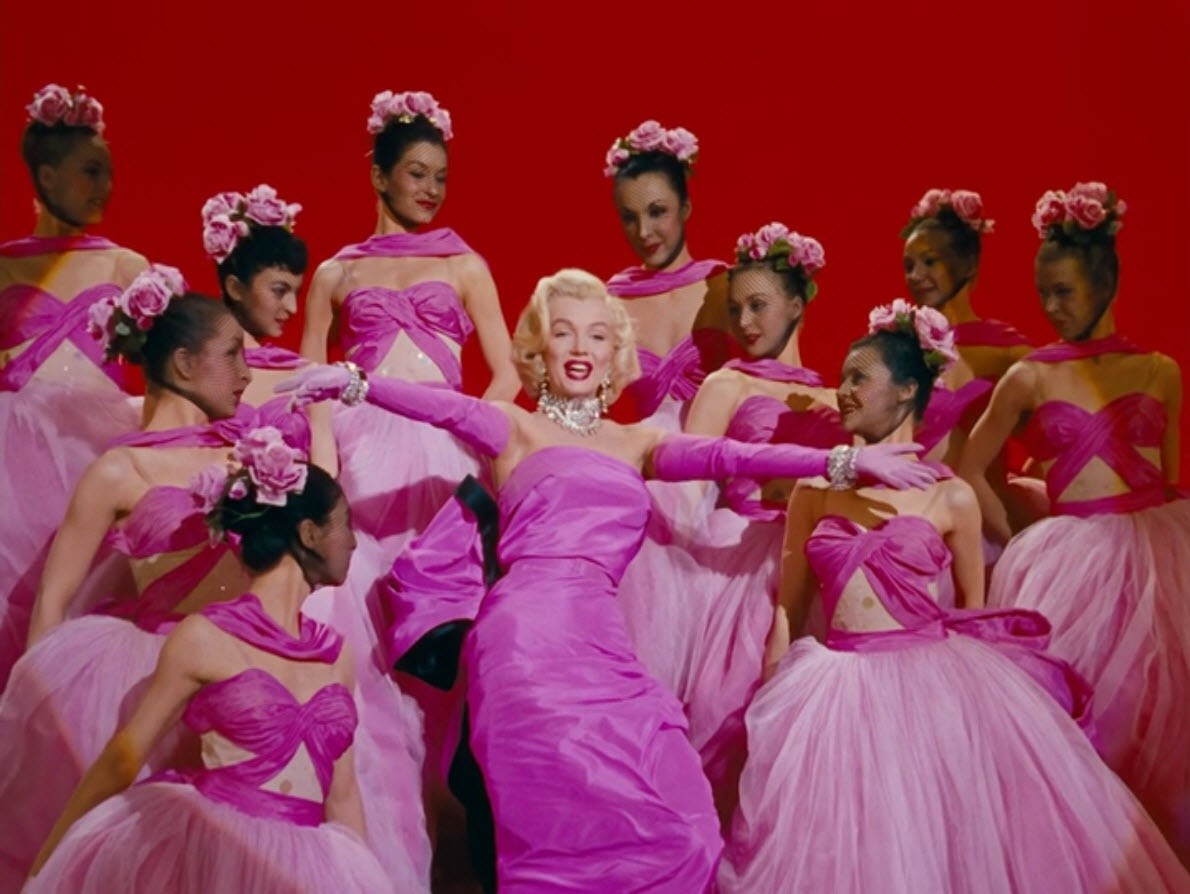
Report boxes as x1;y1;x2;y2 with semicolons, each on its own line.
352;369;370;405
332;357;360;405
829;443;850;492
846;447;860;491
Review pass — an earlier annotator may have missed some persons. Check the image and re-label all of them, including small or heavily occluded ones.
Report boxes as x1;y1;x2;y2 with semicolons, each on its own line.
0;84;1190;894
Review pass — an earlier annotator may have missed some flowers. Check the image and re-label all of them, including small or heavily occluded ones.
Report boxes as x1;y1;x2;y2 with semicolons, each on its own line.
734;222;828;300
367;89;455;144
868;299;961;373
911;188;996;236
25;72;103;130
604;120;699;174
1031;182;1127;249
190;424;308;552
87;263;187;367
201;187;303;267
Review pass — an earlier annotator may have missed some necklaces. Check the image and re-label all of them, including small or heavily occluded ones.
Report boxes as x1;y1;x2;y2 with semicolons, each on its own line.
534;395;602;434
